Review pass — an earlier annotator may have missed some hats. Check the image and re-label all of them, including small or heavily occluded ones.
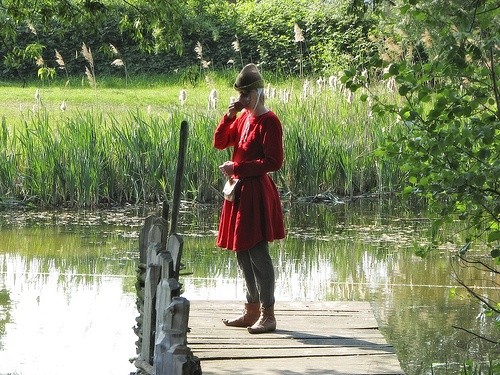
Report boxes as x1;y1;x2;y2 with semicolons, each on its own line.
233;63;264;91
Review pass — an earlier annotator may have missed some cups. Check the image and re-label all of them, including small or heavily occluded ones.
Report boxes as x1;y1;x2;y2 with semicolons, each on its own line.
234;93;250;112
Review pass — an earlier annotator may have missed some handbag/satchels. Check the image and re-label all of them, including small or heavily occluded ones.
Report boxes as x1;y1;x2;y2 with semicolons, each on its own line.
223;178;240;202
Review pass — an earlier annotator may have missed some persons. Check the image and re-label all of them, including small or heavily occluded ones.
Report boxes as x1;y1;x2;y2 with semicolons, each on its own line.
212;63;285;334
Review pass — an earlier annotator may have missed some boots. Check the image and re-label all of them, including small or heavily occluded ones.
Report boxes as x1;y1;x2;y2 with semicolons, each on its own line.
221;302;262;326
247;299;276;333
